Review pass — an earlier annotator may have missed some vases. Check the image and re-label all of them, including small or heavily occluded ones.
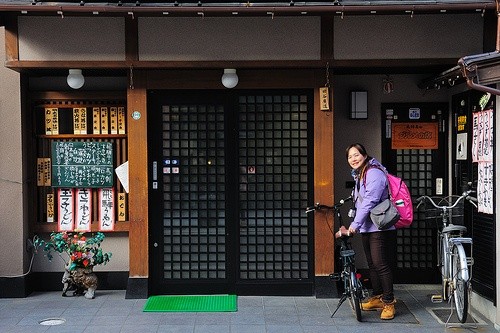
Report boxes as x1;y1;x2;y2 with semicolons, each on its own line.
61;262;99;299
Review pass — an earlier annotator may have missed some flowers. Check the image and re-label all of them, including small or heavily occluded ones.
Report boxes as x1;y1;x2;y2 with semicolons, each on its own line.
33;227;114;270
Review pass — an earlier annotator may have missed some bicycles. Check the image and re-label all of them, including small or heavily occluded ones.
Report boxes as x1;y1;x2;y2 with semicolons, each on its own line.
414;189;479;331
305;190;368;322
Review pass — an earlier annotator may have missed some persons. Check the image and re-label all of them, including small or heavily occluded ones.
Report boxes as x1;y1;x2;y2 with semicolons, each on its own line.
345;142;397;319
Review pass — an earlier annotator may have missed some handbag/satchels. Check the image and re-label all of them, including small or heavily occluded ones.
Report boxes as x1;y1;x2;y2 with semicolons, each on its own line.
370;198;400;230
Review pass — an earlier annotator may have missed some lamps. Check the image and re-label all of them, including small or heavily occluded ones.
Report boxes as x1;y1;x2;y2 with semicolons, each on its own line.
351;90;368;120
221;68;240;89
67;67;86;89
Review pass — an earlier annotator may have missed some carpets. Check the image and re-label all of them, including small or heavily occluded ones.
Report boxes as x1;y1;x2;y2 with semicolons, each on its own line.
142;296;239;313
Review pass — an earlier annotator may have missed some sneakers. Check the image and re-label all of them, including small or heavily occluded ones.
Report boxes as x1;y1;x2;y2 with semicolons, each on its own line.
359;294;386;311
380;304;396;319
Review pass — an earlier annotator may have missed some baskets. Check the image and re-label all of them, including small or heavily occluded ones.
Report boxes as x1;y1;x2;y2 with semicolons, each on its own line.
424;195;465;229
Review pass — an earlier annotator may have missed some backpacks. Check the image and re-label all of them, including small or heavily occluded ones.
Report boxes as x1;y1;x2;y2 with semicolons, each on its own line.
362;163;413;228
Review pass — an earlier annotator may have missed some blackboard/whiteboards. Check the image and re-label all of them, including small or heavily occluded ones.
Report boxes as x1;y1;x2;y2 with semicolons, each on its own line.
50;139;112;189
